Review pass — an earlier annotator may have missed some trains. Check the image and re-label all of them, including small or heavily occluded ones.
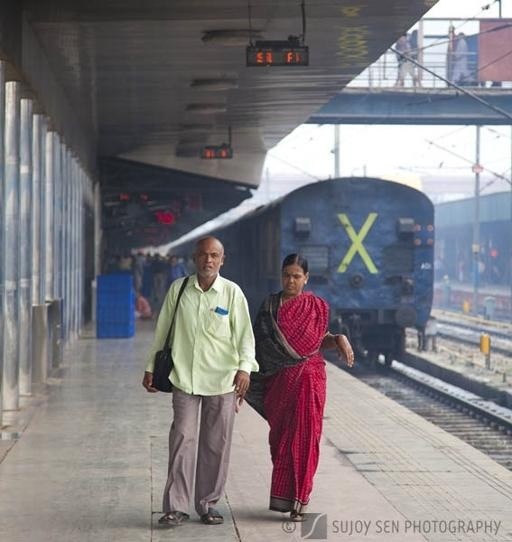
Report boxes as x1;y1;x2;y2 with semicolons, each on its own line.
167;176;434;372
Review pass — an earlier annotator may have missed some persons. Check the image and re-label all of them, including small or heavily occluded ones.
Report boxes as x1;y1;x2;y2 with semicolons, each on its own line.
395;30;474;88
142;236;259;525
235;254;355;522
102;250;191;319
435;253;505;285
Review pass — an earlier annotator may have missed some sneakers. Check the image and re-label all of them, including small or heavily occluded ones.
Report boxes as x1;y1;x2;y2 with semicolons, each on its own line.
290;510;303;521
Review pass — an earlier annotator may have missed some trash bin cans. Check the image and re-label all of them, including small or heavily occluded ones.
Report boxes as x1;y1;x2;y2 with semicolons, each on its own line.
96;271;136;338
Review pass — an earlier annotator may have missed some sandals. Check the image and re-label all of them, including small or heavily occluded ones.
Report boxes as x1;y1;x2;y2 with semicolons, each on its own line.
201;507;223;525
158;511;190;524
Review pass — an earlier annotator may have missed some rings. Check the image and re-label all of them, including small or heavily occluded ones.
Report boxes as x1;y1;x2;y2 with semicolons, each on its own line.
351;352;354;355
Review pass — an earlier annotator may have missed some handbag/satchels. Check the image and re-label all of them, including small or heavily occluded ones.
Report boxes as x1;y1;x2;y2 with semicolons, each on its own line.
151;350;173;392
